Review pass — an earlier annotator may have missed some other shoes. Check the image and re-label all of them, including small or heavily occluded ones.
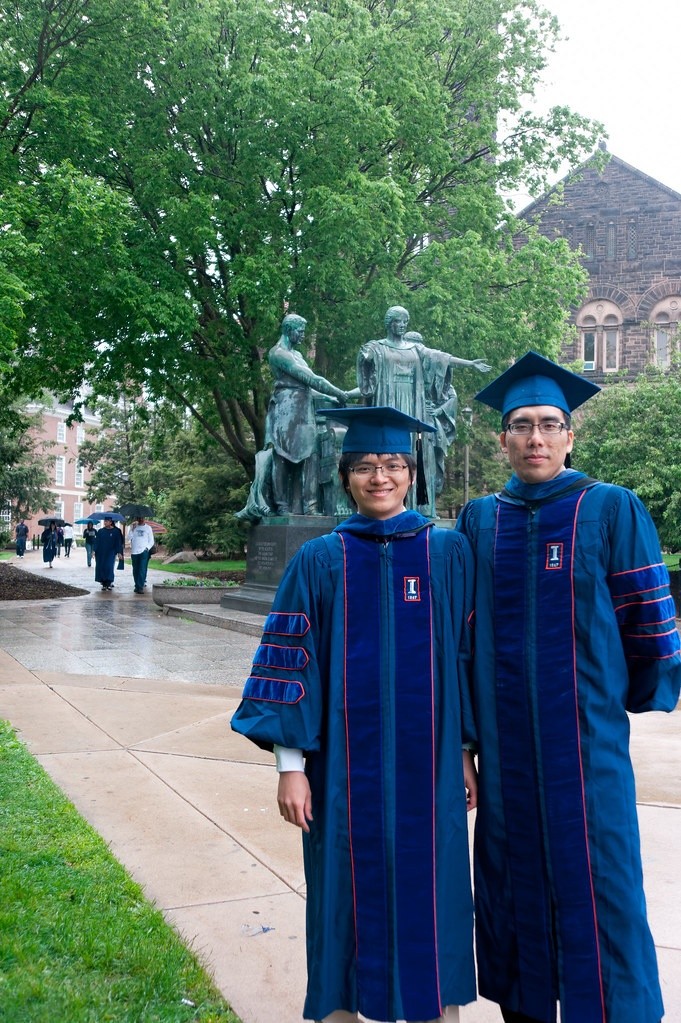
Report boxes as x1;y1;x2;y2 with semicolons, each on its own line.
111;584;114;587
17;556;19;558
138;589;144;594
107;586;112;590
144;582;147;587
101;587;106;591
50;566;52;568
134;588;138;592
88;564;91;567
20;556;24;559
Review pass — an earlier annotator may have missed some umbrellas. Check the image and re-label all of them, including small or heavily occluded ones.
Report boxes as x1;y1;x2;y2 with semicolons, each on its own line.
132;520;167;532
75;519;100;526
118;503;157;517
38;517;65;527
87;512;125;521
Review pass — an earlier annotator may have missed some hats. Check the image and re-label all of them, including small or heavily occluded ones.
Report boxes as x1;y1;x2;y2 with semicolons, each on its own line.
474;350;602;468
316;406;439;506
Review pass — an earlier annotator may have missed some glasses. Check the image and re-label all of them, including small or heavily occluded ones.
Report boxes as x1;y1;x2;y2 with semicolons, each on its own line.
349;463;409;477
504;422;568;435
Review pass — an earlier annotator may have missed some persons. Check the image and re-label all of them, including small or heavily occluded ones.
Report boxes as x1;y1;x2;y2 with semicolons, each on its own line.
15;518;28;558
231;407;480;1023
41;522;64;568
63;524;74;557
92;517;123;591
127;517;154;594
83;522;97;567
455;351;681;1023
233;306;492;520
